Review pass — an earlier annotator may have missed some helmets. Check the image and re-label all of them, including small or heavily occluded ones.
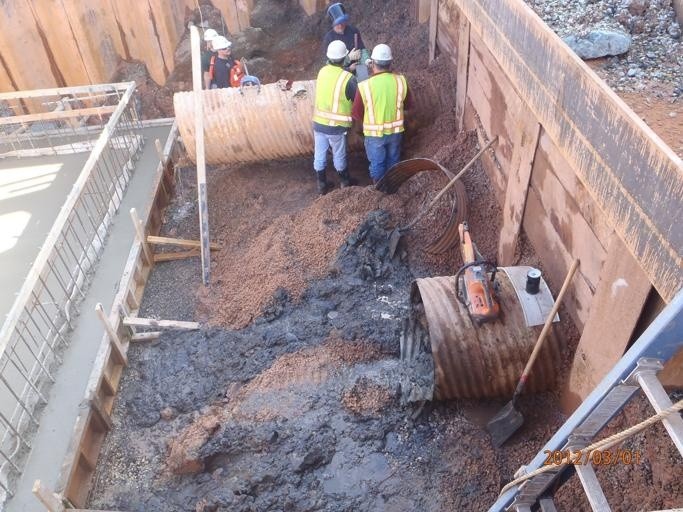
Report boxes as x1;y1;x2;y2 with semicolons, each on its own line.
212;36;231;50
204;29;218;41
326;40;349;59
372;44;393;60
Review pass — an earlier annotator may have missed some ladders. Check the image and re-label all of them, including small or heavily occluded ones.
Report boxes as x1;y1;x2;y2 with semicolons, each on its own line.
486;287;683;512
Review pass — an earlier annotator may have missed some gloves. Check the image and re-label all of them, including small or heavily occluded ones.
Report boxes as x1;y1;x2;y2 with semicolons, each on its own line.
349;47;373;71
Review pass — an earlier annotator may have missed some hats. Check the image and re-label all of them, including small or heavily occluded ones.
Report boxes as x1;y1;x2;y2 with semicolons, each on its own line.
328;3;349;26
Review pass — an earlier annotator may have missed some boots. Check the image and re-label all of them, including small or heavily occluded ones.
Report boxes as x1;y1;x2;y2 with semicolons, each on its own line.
338;166;356;188
316;167;334;193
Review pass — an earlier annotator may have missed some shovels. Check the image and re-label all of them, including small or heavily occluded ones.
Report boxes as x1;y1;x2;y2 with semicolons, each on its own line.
387;135;498;261
486;260;581;448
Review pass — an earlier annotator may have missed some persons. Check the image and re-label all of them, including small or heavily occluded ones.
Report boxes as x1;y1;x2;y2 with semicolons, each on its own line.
201;29;248;88
351;44;415;185
311;40;358;195
321;3;366;65
